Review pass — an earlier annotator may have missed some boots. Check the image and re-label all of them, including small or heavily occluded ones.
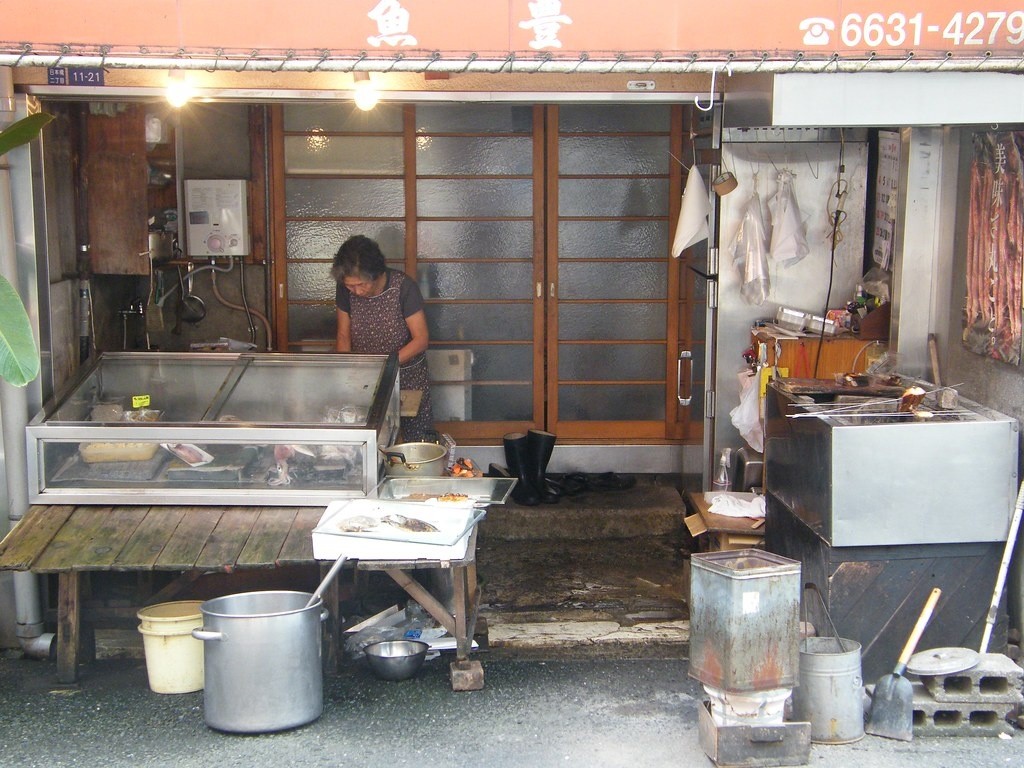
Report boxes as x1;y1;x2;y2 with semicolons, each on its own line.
527;428;557;505
503;433;541;507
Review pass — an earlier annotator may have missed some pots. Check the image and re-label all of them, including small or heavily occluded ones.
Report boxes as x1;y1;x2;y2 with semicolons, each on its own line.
380;430;447;477
149;225;174;260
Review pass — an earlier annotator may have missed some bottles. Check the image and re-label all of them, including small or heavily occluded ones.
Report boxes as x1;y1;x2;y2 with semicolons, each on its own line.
712;451;733;493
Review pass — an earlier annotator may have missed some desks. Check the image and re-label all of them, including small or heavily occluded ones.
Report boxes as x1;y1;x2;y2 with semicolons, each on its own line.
0;465;477;682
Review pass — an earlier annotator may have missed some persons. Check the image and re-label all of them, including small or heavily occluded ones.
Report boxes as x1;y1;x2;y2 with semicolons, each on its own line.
331;234;436;444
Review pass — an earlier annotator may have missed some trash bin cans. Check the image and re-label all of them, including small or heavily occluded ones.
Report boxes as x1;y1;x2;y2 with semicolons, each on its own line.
687;548;802;694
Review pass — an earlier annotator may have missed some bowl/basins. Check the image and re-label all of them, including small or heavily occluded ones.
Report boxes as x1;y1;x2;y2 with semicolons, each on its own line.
362;640;432;681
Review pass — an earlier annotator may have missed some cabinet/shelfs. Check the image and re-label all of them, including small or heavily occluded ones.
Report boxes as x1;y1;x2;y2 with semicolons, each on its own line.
83;107;181;278
747;328;874;376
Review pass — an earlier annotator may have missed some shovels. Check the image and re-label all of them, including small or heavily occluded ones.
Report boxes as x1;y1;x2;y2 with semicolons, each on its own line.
865;587;941;741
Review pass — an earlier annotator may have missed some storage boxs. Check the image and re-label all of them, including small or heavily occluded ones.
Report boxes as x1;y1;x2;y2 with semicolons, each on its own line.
683;514;766;551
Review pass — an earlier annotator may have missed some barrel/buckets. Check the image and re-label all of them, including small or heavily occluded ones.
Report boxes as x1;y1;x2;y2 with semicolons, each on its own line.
191;590;325;734
686;548;805;698
136;600;204;694
793;636;866;745
